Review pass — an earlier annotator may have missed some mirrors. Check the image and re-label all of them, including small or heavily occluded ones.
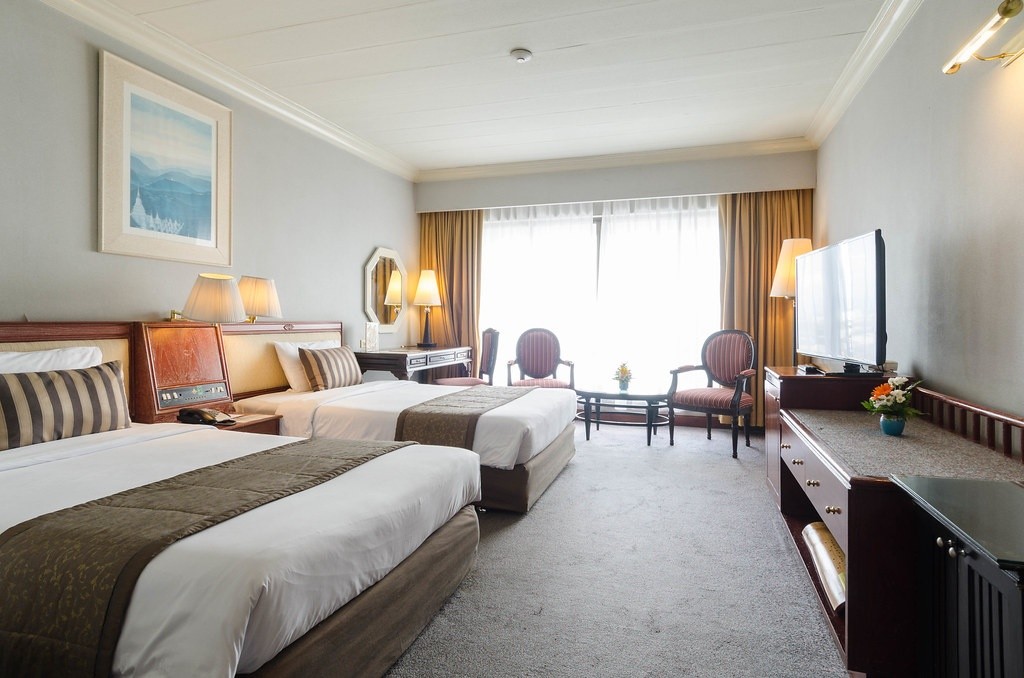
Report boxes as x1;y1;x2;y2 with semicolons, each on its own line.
364;247;408;333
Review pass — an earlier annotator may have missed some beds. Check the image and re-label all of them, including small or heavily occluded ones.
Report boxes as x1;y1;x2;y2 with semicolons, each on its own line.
0;321;483;678
178;319;578;515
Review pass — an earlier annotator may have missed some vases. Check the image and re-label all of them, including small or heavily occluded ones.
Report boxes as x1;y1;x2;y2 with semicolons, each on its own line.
880;413;905;436
618;379;629;390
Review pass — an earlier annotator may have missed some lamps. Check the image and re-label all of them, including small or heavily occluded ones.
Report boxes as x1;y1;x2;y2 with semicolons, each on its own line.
412;270;441;348
769;238;813;367
238;275;283;324
383;270;402;316
165;273;247;323
942;0;1024;74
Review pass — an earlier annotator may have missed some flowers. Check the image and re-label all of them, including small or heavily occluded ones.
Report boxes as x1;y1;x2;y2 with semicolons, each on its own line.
611;360;634;383
860;377;930;423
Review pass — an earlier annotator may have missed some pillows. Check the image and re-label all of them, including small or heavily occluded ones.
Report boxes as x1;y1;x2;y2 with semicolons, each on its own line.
0;360;133;451
272;340;341;393
298;344;365;392
0;347;102;373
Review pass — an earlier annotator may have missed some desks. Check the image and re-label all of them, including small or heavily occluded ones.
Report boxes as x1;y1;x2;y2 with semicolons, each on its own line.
574;382;667;446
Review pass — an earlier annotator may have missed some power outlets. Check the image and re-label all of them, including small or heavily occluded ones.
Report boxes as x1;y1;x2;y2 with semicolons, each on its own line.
360;339;366;348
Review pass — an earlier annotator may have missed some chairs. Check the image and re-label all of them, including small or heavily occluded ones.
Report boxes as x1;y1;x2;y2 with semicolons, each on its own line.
507;328;575;389
666;329;758;460
433;328;500;385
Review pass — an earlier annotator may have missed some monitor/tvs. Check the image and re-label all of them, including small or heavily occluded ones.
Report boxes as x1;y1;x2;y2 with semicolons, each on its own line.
796;228;888;377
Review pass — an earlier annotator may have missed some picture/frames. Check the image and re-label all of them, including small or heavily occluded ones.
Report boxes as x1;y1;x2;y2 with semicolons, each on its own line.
97;47;233;268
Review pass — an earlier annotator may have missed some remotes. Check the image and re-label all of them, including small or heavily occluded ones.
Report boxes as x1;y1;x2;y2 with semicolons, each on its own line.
797;363;817;372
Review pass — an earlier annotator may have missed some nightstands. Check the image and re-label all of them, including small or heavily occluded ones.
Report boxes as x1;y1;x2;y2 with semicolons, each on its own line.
353;345;473;381
133;321;283;435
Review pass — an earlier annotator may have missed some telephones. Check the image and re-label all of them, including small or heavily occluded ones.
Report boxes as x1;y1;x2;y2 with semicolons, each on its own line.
176;407;236;426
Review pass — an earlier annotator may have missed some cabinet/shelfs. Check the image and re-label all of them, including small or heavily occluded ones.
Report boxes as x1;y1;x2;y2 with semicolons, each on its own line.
915;507;1024;678
764;365;912;554
